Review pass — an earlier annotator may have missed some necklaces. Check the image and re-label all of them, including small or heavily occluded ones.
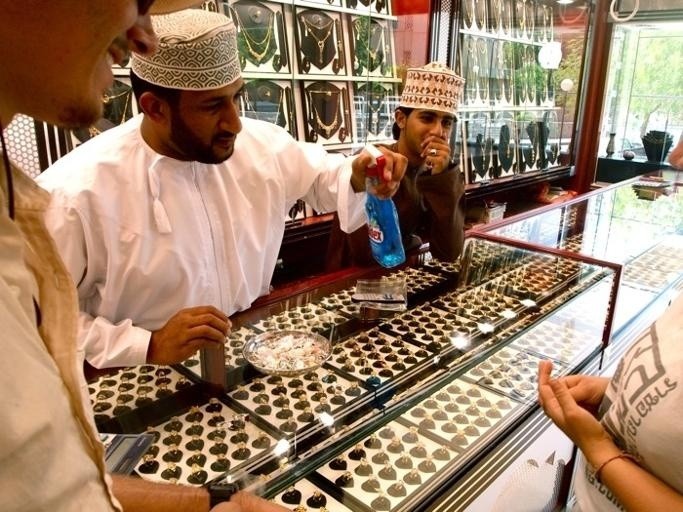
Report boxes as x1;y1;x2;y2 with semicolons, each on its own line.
300;15;336;64
456;1;565;184
242;89;284;126
365;97;386;134
305;89;345;137
351;20;385;65
230;7;275;64
82;89;130;139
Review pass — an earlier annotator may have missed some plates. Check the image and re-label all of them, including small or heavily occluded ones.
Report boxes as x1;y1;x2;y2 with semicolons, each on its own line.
243;329;333;376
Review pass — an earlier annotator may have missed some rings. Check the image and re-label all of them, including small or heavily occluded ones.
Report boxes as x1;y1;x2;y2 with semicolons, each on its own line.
429;148;437;157
90;236;617;512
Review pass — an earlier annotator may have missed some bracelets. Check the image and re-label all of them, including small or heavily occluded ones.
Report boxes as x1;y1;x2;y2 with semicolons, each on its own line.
204;480;244;512
594;451;640;484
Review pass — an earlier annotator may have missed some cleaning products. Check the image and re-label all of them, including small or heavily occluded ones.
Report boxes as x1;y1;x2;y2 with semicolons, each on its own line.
363;141;406;268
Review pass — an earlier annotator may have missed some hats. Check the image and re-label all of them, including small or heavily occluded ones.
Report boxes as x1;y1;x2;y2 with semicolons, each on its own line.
146;0;206;15
399;61;466;121
130;8;241;92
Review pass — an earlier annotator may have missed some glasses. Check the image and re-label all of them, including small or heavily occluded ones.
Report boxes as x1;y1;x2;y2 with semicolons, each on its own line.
135;0;156;16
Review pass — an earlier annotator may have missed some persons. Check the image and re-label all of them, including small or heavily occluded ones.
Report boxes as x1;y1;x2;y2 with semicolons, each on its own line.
317;58;468;276
533;286;683;512
24;8;411;375
0;1;304;512
667;131;683;171
